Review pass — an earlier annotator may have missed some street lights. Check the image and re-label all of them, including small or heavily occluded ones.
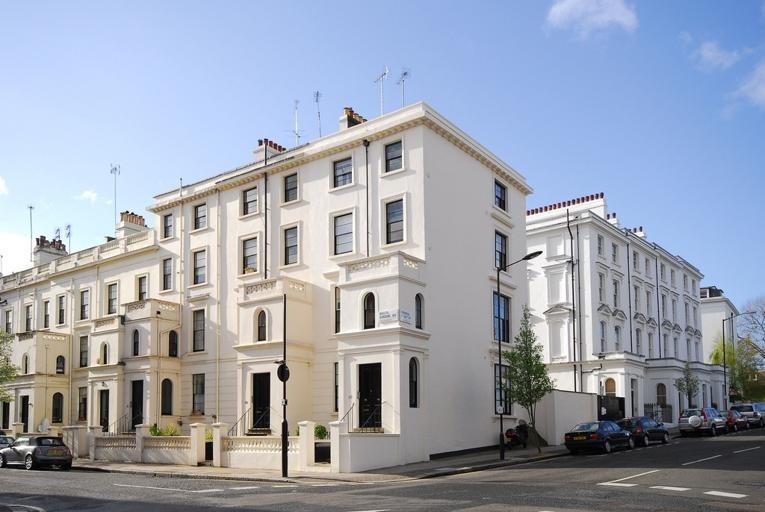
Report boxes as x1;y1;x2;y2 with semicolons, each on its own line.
722;310;759;412
497;248;544;460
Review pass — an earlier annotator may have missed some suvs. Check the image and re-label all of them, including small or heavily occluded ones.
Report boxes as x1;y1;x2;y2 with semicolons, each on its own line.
731;402;765;427
678;408;730;436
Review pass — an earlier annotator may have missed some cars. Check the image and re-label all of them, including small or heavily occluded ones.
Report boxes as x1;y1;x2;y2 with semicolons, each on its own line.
565;420;636;453
0;436;72;471
0;435;16;449
616;415;671;445
719;410;751;431
